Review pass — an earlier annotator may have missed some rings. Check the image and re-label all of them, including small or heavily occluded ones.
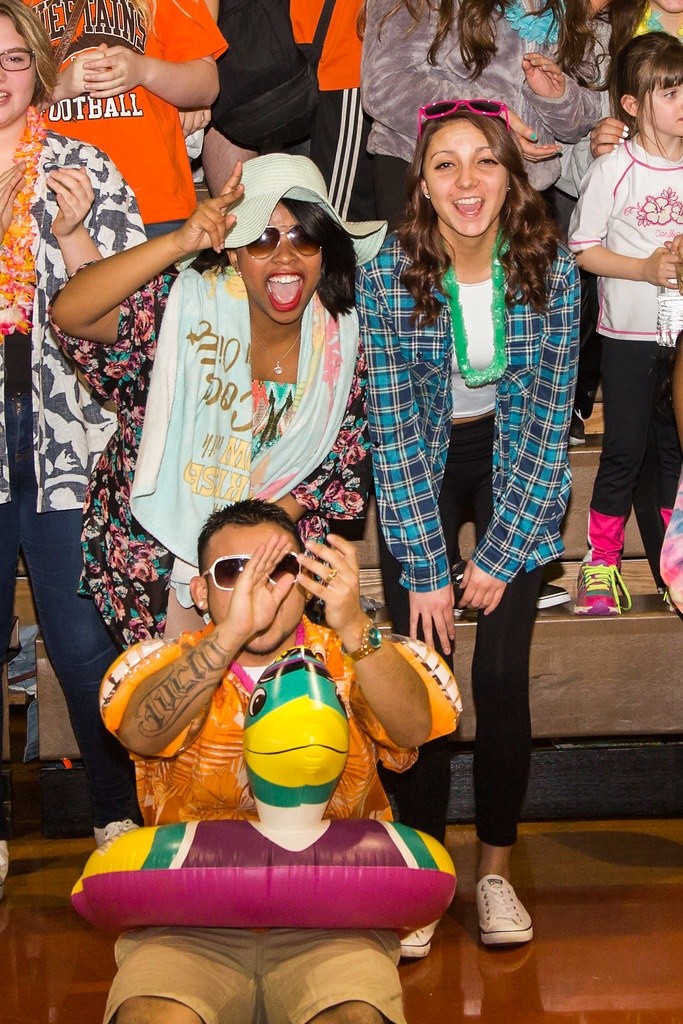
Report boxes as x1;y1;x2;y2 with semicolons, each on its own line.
326;570;337;582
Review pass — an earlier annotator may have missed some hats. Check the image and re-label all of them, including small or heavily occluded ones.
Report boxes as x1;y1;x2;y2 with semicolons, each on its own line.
175;153;388;273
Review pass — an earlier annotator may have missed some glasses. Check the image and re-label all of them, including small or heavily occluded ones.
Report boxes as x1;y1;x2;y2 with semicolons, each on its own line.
0;51;35;71
202;551;301;591
246;226;323;258
418;100;509;141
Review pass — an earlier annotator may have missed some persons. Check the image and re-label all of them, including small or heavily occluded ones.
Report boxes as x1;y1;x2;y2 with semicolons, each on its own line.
24;0;683;620
53;149;375;652
104;498;463;1023
0;0;143;881
356;96;582;961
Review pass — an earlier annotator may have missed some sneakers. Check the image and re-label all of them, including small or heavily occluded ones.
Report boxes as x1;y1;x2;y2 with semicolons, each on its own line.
536;582;572;608
400;918;440;957
94;819;140;849
476;874;532;944
450;561;479;618
574;560;631;614
0;840;9;901
568;409;585;446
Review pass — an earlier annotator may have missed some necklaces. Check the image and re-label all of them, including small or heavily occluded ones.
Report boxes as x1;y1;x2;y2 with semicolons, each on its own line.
247;322;302;374
441;227;509;387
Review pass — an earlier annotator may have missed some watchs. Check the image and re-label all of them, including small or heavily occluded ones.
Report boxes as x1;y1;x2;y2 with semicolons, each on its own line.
349;624;383;662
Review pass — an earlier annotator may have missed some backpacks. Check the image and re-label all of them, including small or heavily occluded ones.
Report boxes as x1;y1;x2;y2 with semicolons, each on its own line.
209;0;337;152
